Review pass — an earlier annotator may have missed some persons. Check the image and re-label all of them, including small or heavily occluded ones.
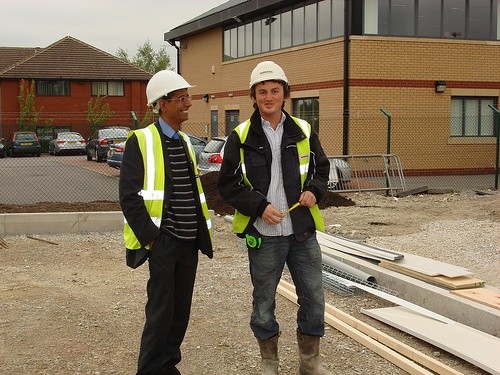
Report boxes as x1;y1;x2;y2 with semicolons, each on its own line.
217;60;330;375
119;68;213;375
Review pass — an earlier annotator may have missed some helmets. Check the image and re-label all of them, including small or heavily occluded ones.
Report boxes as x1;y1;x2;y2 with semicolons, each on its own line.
146;69;196;113
250;60;289;89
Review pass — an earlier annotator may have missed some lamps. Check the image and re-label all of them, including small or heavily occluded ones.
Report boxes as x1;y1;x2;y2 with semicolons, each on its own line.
265;18;277;25
233;15;242;23
203;94;208;103
436;80;447;93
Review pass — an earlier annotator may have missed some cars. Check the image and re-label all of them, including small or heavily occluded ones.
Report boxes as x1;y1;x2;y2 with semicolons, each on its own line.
86;126;132;162
190;135;211;164
8;131;41;158
49;132;87;156
0;137;7;158
107;133;210;170
196;135;352;190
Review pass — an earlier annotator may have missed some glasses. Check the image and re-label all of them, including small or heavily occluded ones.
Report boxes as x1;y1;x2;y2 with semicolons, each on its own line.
164;95;192;104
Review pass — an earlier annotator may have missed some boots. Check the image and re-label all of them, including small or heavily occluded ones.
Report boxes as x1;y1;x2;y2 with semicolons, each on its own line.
296;328;334;375
255;331;282;375
